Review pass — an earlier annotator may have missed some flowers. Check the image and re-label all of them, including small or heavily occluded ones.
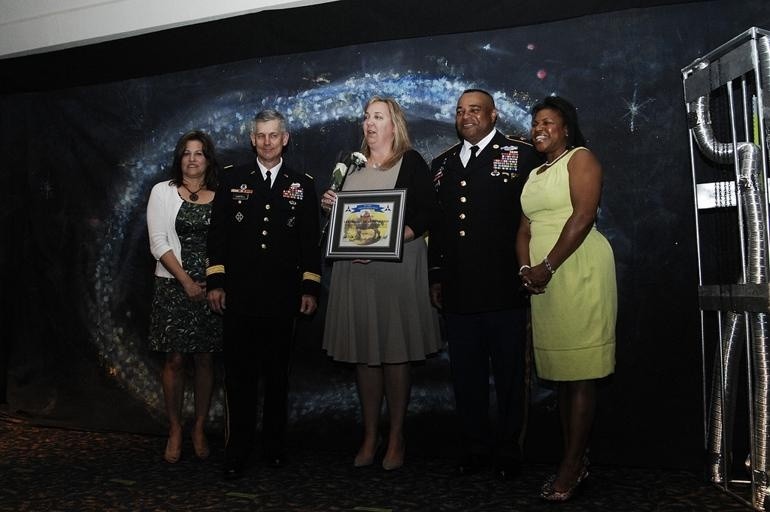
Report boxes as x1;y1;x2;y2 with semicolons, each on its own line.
317;151;367;247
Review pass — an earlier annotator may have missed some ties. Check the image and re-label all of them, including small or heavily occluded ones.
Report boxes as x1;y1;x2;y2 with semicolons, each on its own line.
265;170;272;190
464;145;480;169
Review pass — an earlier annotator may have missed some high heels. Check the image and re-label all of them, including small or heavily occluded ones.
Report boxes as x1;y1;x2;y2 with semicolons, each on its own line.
190;426;211;461
164;430;183;464
542;452;592;489
353;434;384;469
539;466;593;504
383;437;406;471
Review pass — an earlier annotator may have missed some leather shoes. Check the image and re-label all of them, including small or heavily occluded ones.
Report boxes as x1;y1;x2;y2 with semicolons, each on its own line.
216;443;246;478
263;439;290;468
449;456;486;483
493;457;530;482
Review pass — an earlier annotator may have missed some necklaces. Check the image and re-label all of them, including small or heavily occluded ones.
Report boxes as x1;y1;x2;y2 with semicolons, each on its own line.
181;181;205;202
545;145;570;167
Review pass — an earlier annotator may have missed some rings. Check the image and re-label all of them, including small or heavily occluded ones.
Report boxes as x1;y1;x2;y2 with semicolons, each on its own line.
523;283;530;287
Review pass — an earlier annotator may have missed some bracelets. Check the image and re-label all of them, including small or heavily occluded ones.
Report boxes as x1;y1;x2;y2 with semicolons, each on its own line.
544;257;556;275
518;265;531;274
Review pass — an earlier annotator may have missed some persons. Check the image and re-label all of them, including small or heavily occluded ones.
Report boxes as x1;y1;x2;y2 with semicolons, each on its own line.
516;95;619;501
146;130;219;464
428;89;544;482
321;95;439;472
205;109;321;481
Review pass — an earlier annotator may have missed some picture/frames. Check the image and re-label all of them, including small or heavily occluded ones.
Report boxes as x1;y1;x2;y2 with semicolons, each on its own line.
325;189;407;263
